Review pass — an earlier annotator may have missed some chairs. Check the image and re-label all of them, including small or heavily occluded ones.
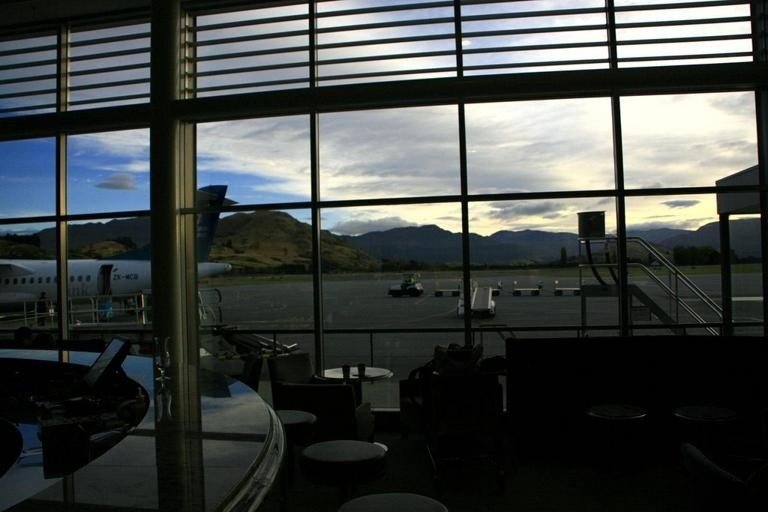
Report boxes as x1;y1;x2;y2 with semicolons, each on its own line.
265;342;504;441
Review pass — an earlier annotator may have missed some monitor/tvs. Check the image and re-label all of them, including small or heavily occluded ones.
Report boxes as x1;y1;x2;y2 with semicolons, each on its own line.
82;335;131;391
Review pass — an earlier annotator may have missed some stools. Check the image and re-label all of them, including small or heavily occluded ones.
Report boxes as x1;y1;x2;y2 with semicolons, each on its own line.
275;407;447;511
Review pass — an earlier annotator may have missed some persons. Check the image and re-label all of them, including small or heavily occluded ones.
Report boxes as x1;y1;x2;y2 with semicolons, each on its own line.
37;290;53;326
137;288;145;323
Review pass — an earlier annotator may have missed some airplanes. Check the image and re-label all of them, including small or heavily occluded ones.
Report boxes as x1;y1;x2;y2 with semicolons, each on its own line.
0;184;236;322
